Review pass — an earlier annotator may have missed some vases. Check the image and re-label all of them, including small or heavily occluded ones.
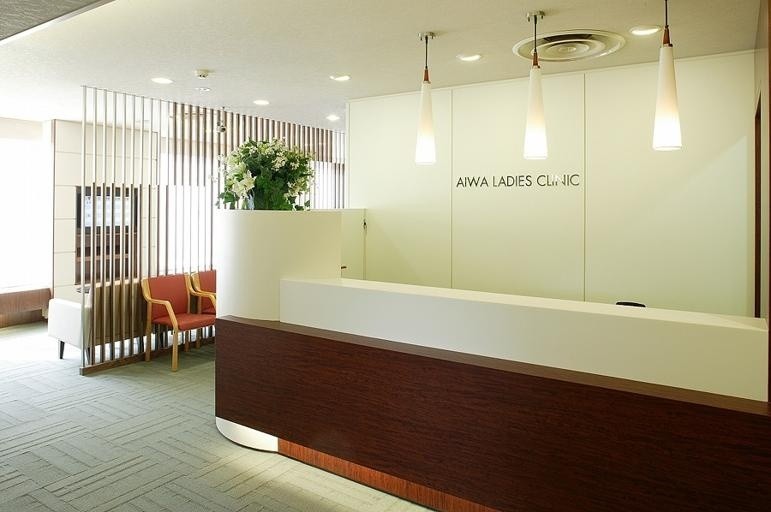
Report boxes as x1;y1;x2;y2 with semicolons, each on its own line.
253;187;281;210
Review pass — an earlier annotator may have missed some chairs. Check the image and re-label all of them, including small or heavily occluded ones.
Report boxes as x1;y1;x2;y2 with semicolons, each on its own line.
191;271;216;348
140;274;215;372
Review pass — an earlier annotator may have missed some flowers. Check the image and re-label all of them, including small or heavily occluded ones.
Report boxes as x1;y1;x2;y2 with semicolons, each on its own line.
213;137;317;210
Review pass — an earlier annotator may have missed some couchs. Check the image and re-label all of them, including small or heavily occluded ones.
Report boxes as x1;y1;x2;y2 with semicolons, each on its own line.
48;278;140;364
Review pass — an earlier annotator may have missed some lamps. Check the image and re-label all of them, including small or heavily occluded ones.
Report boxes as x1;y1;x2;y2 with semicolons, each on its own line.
414;36;436;164
652;0;683;152
523;14;547;158
